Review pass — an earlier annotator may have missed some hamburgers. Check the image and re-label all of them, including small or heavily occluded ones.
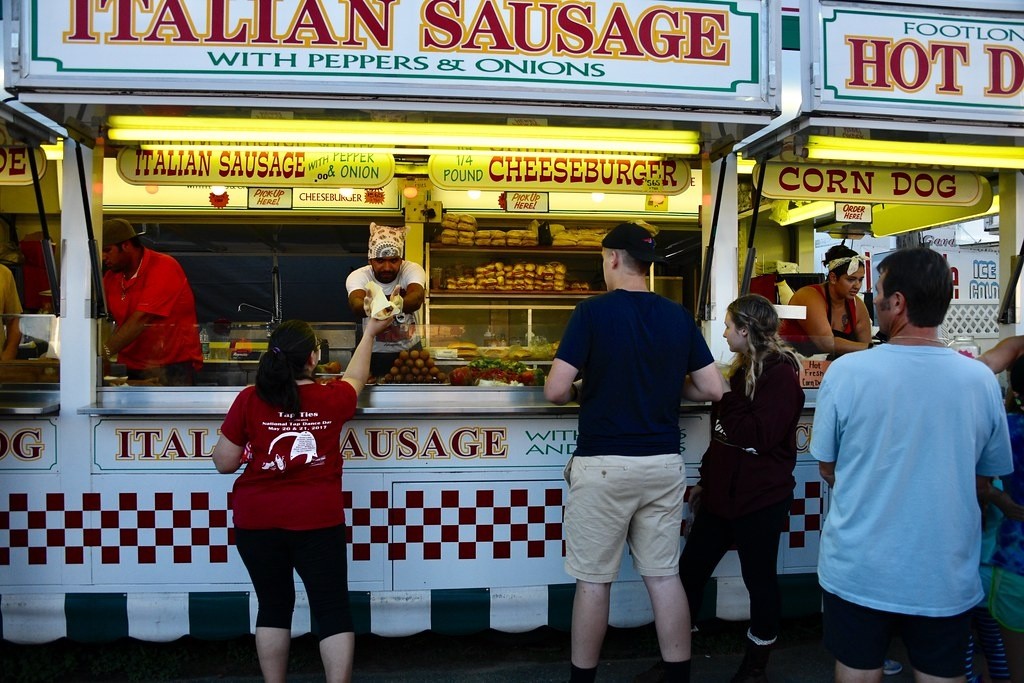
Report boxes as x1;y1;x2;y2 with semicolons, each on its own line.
513;350;533;360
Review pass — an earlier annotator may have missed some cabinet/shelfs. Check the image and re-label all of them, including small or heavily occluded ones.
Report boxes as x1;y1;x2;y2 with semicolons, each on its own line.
420;241;655;368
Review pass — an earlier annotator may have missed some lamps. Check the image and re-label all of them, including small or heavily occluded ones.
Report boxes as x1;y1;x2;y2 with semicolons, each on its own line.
90;115;714;157
792;133;1024;169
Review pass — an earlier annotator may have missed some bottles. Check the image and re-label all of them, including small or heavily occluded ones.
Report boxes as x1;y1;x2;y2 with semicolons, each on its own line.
949;335;981;359
199;328;210;360
483;326;535;347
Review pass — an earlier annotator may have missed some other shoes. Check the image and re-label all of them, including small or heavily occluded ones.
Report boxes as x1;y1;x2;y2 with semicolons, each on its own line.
883;658;903;675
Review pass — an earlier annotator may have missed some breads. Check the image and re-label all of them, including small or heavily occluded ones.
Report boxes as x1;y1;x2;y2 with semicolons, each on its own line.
440;213;537;246
446;342;480;359
549;223;606;246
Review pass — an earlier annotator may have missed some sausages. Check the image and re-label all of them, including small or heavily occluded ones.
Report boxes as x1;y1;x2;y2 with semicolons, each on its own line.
385;349;446;382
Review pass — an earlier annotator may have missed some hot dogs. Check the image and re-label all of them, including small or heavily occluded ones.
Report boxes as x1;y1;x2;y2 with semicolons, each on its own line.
446;261;589;292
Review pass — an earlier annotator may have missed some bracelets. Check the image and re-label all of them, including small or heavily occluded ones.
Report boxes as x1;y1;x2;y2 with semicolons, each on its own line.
868;343;873;348
104;344;110;361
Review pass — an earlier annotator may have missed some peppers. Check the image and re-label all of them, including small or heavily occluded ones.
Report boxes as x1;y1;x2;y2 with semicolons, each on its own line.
450;368;535;387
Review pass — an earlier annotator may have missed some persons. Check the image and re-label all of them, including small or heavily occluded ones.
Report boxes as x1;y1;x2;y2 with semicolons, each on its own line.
212;315;394;683
346;223;426;377
0;264;22;361
544;222;723;683
779;245;878;361
680;293;806;683
974;337;1024;408
810;249;1013;683
990;359;1024;683
101;218;203;387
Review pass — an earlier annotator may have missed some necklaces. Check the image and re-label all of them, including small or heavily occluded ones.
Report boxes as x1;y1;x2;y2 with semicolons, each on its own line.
890;336;942;346
121;261;141;300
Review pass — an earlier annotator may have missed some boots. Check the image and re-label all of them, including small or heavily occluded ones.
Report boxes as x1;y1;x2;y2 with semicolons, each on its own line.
630;627;699;683
727;627;778;683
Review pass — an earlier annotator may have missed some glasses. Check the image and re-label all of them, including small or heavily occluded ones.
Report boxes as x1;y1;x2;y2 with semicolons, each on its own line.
313;338;322;352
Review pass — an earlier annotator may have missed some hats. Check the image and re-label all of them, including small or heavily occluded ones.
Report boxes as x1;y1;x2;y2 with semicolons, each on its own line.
601;223;669;265
367;222;407;259
103;218;145;246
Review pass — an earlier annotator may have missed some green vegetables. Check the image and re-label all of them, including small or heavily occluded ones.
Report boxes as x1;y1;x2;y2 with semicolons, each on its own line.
469;359;526;373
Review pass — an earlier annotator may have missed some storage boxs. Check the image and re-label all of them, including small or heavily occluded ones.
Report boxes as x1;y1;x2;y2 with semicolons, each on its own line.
756;260;799;274
